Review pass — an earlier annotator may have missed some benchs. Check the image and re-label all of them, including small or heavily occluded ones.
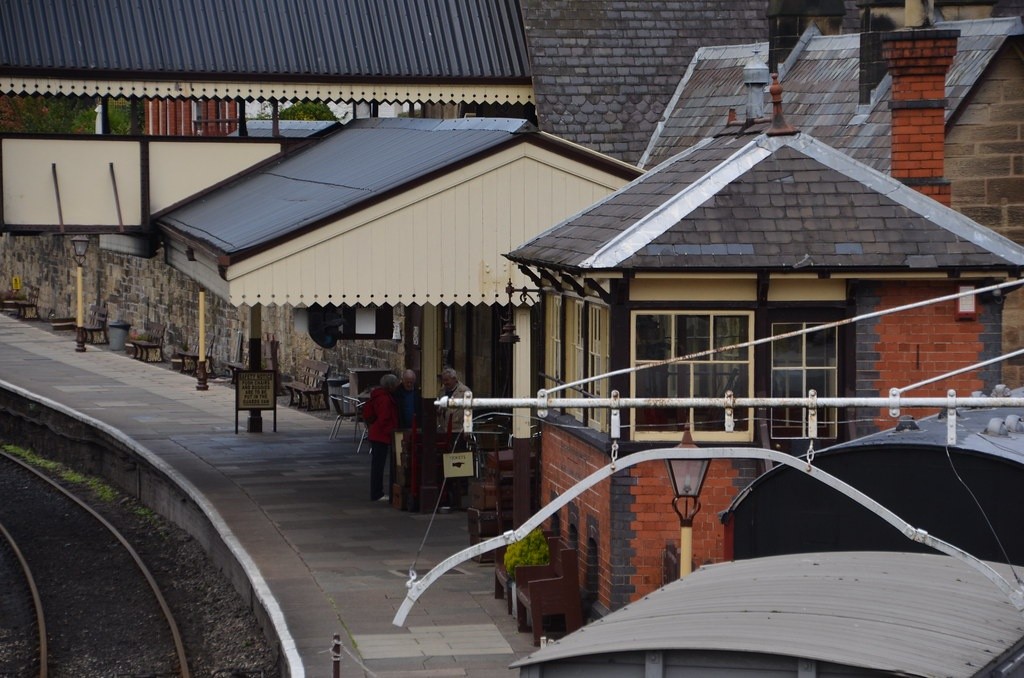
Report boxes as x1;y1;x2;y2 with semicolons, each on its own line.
514;537;585;646
81;305;108;345
285;359;331;412
225;343;249;384
467;508;513;565
178;334;216;376
495;530;552;614
15;286;41;321
129;323;166;362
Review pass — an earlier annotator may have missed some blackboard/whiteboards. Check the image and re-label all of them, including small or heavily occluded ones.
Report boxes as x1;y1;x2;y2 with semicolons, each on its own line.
235;370;276;410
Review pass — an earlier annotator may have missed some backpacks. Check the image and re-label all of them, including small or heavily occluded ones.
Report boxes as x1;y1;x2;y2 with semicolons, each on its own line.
362;392;379;425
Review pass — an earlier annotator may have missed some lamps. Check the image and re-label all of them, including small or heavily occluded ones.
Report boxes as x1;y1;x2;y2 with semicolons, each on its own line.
499;277;540;343
392;321;402;340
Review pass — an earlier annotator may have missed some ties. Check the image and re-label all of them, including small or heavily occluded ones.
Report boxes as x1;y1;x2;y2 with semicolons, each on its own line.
443;386;457;418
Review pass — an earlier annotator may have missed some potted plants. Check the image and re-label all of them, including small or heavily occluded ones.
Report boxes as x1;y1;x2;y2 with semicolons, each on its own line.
504;527;550;626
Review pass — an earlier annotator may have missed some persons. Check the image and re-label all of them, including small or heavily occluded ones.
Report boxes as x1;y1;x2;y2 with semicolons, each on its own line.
436;368;472;507
361;375;397;501
392;369;424;433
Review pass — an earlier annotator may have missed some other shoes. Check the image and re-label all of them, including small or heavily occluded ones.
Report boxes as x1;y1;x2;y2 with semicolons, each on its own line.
377;494;389;502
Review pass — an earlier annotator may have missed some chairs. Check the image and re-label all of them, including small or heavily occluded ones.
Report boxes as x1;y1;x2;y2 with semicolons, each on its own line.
329;394;362;440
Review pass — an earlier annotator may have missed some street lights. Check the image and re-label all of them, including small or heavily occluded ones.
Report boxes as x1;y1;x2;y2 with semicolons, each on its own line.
71;235;89;352
665;419;714;581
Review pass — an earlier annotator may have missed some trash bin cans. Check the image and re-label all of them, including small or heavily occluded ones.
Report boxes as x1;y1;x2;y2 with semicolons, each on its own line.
326;379;350;416
342;382;349;415
